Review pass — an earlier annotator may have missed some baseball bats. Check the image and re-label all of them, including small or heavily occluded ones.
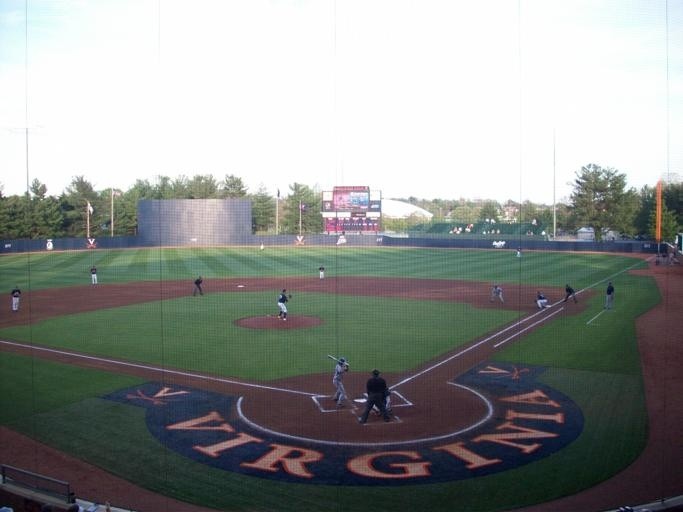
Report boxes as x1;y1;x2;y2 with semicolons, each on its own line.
327;354;349;366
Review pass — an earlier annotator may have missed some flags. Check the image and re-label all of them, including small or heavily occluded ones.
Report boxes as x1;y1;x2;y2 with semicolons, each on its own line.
278;189;281;197
112;190;120;196
298;202;304;211
88;203;94;214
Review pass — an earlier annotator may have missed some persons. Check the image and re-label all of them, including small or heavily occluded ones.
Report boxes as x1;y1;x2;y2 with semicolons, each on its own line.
491;285;504;303
333;358;349;407
536;291;548;309
91;266;97;285
604;282;614;309
564;284;577;303
278;290;287;321
517;246;521;257
193;276;203;296
11;286;21;312
319;265;324;280
358;370;390;423
